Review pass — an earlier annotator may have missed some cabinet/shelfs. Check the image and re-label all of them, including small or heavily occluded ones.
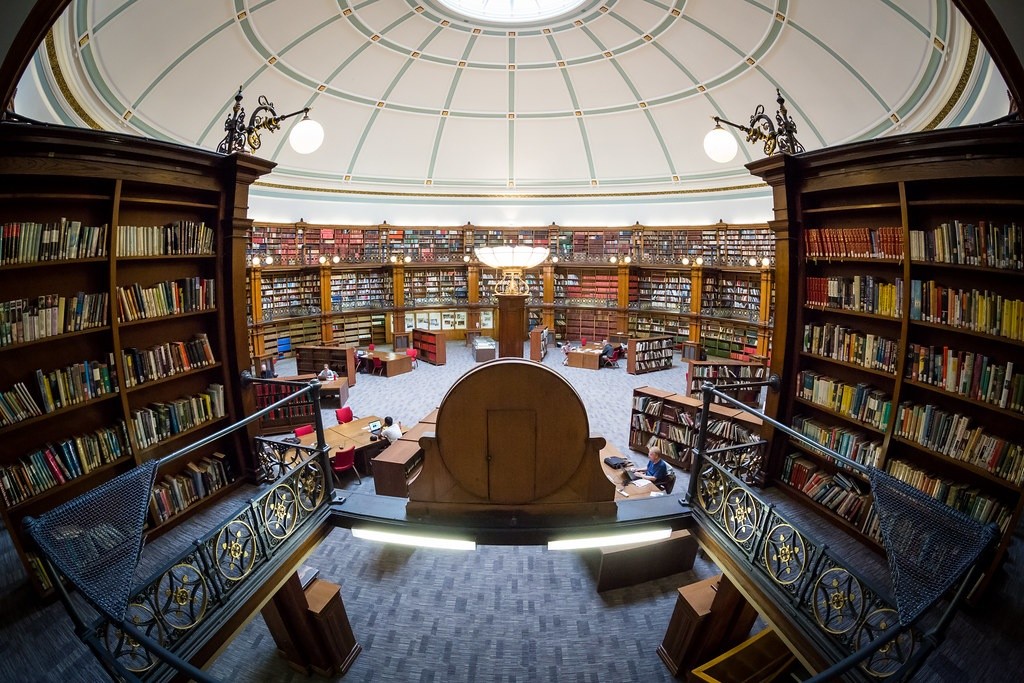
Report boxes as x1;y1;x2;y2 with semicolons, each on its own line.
0;95;1024;683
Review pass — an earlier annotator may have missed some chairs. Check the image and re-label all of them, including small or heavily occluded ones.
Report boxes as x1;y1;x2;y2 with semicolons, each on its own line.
369;344;376;350
654;460;677;495
406;348;419;370
371;357;384;377
604;350;619;370
334;407;356;424
331;445;362;487
292;423;314;438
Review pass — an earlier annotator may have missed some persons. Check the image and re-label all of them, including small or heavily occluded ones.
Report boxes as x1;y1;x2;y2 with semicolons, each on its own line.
600;339;613;365
260;364;278;378
318;364;334;377
353;347;368;373
701;345;706;360
378;417;402;445
629;445;667;483
565;342;571;358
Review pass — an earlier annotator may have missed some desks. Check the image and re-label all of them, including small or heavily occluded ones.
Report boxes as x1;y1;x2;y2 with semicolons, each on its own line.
265;378;350;409
273;415;412;472
567;341;619;370
590;435;663;502
357;348;412;378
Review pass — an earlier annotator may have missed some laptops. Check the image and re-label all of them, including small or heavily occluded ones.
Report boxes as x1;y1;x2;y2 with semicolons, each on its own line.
318;376;326;381
368;419;382;435
620;464;643;480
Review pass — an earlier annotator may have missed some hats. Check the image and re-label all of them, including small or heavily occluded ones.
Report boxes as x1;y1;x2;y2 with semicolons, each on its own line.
324;364;328;368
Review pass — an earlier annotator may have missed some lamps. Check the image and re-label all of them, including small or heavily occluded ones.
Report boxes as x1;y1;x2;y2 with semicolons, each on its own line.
701;87;808;163
214;82;327;156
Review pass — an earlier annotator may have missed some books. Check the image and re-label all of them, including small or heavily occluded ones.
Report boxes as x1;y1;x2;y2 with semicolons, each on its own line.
1;212;1024;560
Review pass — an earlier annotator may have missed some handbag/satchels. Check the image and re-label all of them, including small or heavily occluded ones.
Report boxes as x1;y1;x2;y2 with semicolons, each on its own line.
604;456;631;469
